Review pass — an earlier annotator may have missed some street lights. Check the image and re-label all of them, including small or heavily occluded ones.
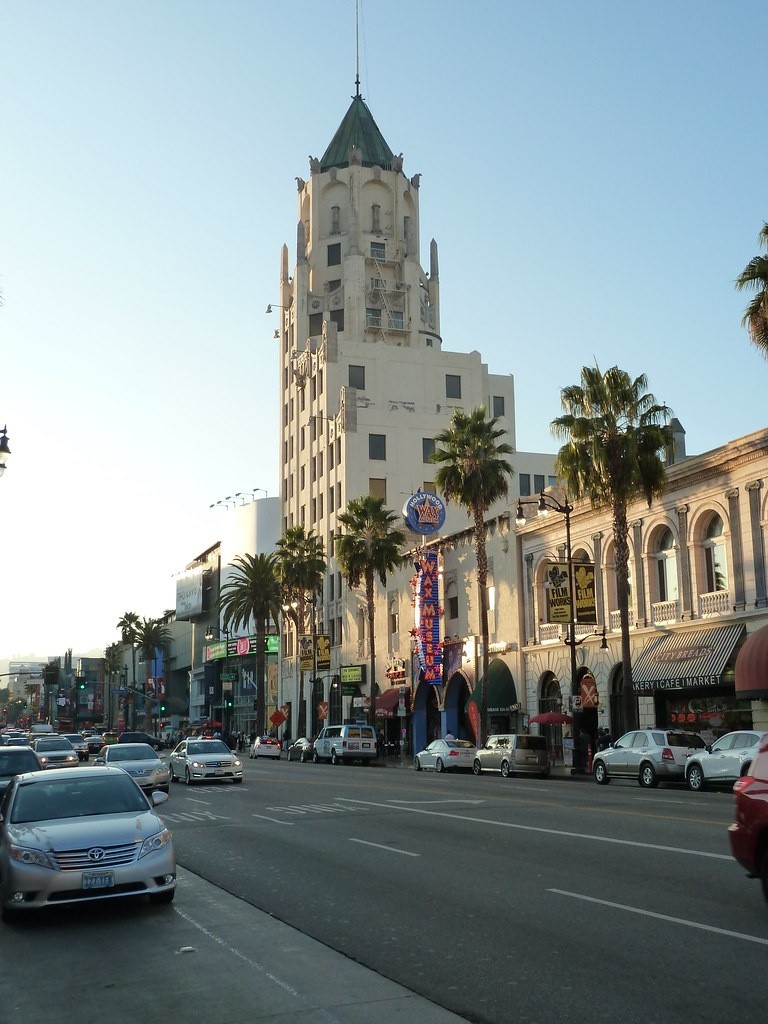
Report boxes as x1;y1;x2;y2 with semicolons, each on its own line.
515;490;609;776
282;591;340;738
205;626;231;731
145;657;157;737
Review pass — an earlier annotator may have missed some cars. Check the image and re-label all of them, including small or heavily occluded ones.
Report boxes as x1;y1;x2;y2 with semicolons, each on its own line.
92;743;170;796
248;736;315;763
728;732;768;903
592;727;707;788
170;736;243;785
0;720;168;806
685;730;768;792
0;766;177;921
472;734;551;778
414;739;479;772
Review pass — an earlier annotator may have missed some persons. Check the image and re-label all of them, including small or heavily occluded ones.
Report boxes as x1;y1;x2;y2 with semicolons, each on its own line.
269;730;275;737
376;729;384;760
445;730;455;740
193;729;247;752
283;735;288;760
598;728;613;752
166;732;182;749
579;728;593;770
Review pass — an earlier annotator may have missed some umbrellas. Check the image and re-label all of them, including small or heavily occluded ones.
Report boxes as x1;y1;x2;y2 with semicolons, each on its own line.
203;720;222;727
528;710;575;766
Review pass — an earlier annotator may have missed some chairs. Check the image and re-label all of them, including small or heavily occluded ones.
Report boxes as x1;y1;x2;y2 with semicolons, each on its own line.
17;791;58;823
79;785;116;811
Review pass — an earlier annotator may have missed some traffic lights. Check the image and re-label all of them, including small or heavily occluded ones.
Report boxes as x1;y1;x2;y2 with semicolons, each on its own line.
160;701;166;715
227;700;233;716
75;677;86;690
23;701;27;709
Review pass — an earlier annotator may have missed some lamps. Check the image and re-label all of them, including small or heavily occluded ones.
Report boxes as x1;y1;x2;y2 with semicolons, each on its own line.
306;416;330;426
266;304;290;313
273;329;280;338
210;489;268;511
290;351;304;359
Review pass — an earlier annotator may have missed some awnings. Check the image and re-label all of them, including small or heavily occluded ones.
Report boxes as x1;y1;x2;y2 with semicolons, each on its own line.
464;658;519;712
631;623;748;690
735;623;768;701
374;686;411;721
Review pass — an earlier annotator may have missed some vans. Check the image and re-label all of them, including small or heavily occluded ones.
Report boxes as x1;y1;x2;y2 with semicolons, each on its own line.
312;725;377;765
